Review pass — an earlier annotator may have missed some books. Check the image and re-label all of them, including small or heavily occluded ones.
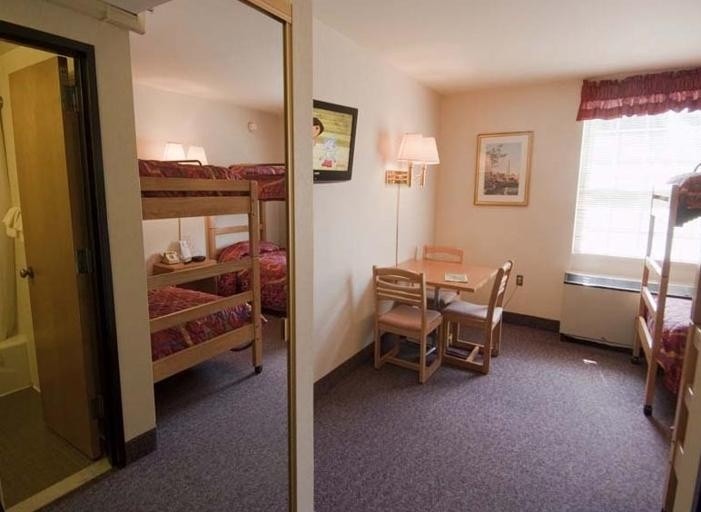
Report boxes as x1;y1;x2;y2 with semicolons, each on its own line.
443;271;468;284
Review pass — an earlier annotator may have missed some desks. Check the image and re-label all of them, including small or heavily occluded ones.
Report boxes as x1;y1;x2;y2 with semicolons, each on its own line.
390;256;499;351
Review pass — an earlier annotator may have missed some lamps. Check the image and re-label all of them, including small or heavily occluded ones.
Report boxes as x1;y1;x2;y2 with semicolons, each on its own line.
385;130;439;189
156;142;211;166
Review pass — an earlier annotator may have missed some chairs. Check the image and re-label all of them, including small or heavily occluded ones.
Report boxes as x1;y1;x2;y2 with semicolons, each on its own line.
439;259;514;374
369;263;444;382
418;242;463;347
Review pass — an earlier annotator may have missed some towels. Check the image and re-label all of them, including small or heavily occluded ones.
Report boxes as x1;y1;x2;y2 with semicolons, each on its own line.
1;205;21;242
14;210;25;242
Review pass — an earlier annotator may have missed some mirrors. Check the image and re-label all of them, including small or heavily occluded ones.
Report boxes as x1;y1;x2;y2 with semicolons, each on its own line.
0;0;317;510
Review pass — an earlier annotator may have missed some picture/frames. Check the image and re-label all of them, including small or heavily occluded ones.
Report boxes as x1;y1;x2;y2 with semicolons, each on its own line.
473;130;532;207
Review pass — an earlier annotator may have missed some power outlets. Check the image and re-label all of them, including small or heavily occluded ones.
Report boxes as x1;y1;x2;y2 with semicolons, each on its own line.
515;274;524;286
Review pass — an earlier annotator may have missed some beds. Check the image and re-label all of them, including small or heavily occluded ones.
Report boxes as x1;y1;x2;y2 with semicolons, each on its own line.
631;259;696;416
649;169;700;229
137;158;289;387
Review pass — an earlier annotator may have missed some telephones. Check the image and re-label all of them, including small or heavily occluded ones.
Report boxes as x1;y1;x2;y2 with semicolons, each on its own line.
162;251;180;265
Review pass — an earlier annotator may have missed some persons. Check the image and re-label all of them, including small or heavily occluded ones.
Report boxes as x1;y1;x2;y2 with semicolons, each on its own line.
312;117;324;168
